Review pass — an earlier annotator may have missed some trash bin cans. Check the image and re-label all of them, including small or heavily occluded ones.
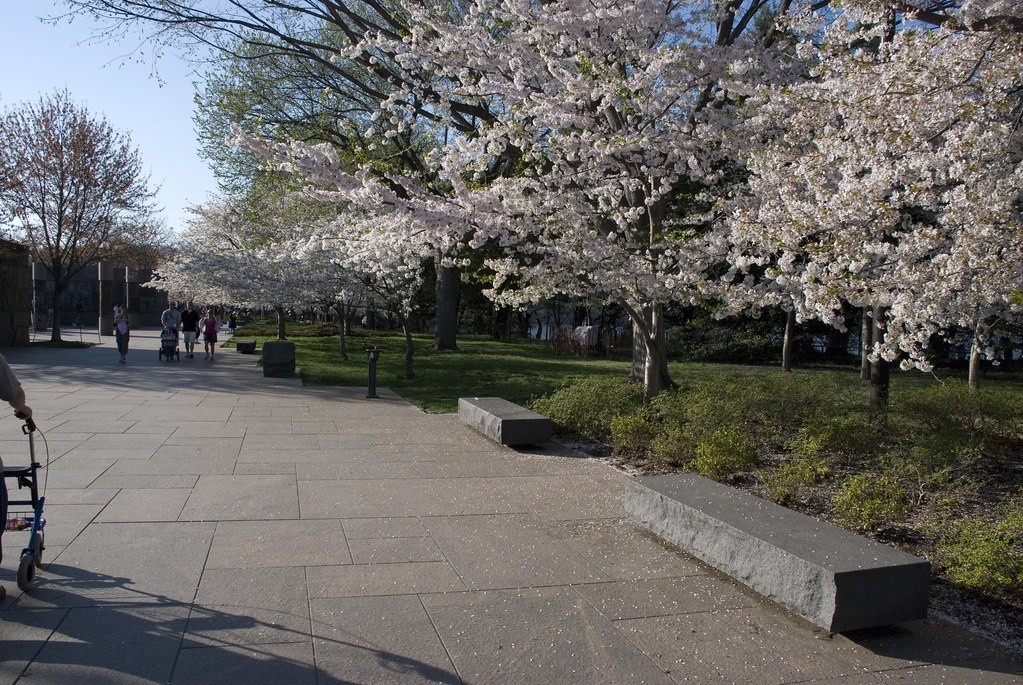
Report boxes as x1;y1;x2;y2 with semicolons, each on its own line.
262;340;296;378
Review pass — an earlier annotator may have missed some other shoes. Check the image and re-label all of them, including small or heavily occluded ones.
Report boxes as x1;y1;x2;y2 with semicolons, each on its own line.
204;354;209;360
119;357;127;363
185;352;193;358
211;354;215;360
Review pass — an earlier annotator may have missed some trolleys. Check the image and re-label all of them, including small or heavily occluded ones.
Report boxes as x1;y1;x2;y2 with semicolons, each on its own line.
0;412;49;591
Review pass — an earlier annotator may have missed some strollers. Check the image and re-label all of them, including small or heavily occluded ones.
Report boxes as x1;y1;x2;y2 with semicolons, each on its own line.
158;325;180;363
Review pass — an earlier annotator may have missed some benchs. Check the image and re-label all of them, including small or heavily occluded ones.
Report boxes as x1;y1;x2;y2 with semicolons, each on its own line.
624;473;932;631
458;396;551;444
236;340;256;354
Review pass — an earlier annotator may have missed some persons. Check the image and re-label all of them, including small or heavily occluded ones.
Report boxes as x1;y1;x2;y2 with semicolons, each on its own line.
0;353;36;602
113;303;130;363
161;301;238;360
